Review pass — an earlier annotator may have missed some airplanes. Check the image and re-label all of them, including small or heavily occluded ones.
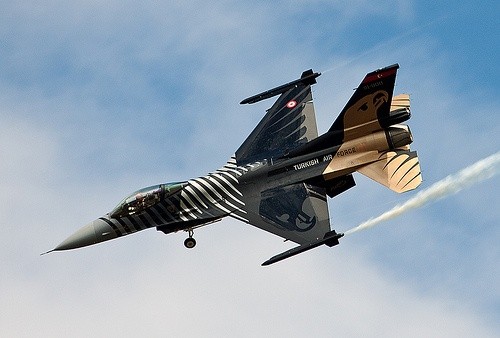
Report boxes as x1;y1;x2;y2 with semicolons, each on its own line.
39;64;457;284
